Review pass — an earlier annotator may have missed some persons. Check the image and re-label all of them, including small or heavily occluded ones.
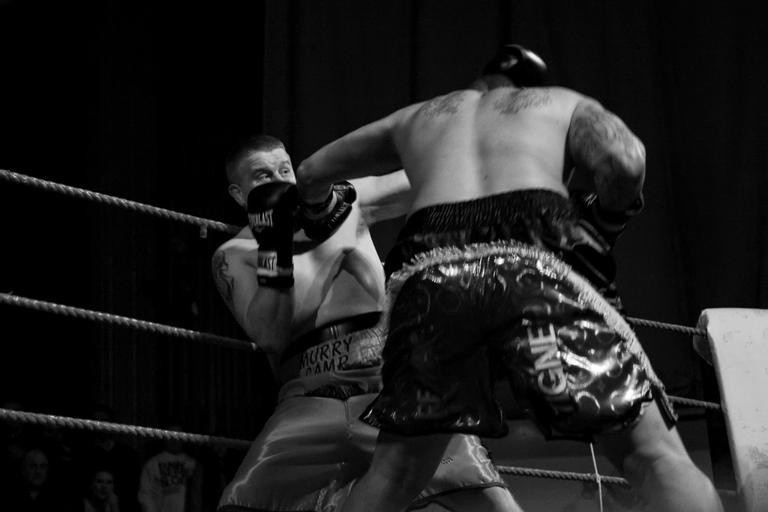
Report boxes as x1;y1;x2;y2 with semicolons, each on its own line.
295;41;726;512
210;128;524;511
0;417;237;512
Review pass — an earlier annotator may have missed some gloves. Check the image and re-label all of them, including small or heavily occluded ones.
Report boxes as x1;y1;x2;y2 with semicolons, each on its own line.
246;179;298;289
576;184;644;242
302;176;355;244
